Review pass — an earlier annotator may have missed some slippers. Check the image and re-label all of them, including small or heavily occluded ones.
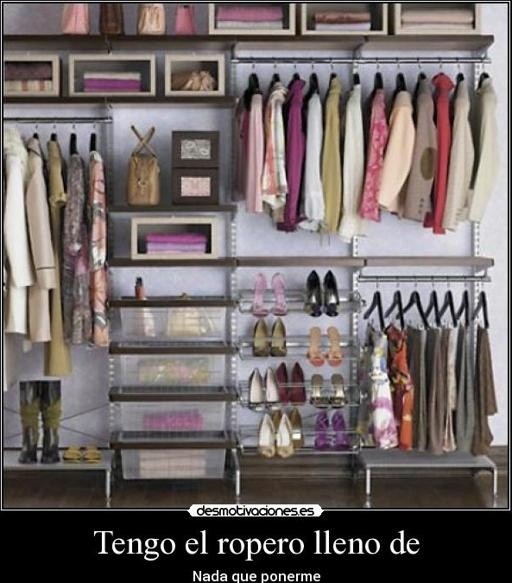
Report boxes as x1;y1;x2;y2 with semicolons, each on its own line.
63;445;101;462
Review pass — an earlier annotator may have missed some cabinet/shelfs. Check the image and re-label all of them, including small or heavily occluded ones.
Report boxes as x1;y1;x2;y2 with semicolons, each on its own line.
2;2;509;511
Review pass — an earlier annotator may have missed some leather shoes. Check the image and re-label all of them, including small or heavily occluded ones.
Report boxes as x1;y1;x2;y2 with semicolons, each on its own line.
252;319;343;366
258;410;348;459
247;270;340;316
248;362;346;412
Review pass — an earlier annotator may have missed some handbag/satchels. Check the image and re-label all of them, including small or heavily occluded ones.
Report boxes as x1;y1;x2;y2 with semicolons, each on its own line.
128;126;159;206
134;278;214;430
62;3;197;35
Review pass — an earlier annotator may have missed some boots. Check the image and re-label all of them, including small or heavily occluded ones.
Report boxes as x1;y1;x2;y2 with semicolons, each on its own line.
18;380;61;464
